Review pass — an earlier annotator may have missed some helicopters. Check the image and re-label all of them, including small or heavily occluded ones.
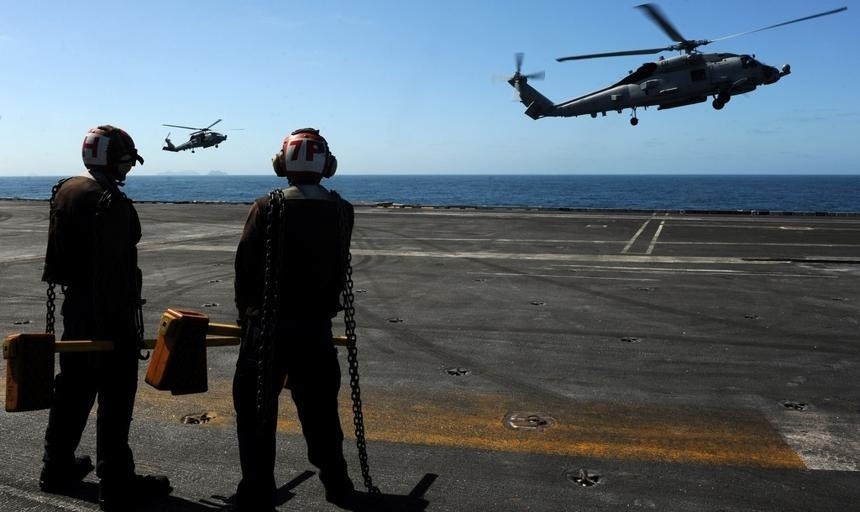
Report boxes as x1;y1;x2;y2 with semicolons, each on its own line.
508;2;848;127
162;119;227;154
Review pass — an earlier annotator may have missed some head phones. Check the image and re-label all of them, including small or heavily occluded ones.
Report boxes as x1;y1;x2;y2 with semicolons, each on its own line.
272;128;337;178
96;125;132;176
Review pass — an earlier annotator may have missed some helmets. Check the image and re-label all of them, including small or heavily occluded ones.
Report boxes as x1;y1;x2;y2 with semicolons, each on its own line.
80;125;144;180
284;127;328;181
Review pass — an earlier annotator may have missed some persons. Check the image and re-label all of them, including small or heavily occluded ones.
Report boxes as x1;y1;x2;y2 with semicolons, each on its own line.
39;125;171;504
231;129;355;506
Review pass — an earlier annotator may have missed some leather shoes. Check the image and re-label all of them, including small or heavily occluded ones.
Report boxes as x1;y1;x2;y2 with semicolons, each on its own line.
39;454;93;491
98;475;174;511
